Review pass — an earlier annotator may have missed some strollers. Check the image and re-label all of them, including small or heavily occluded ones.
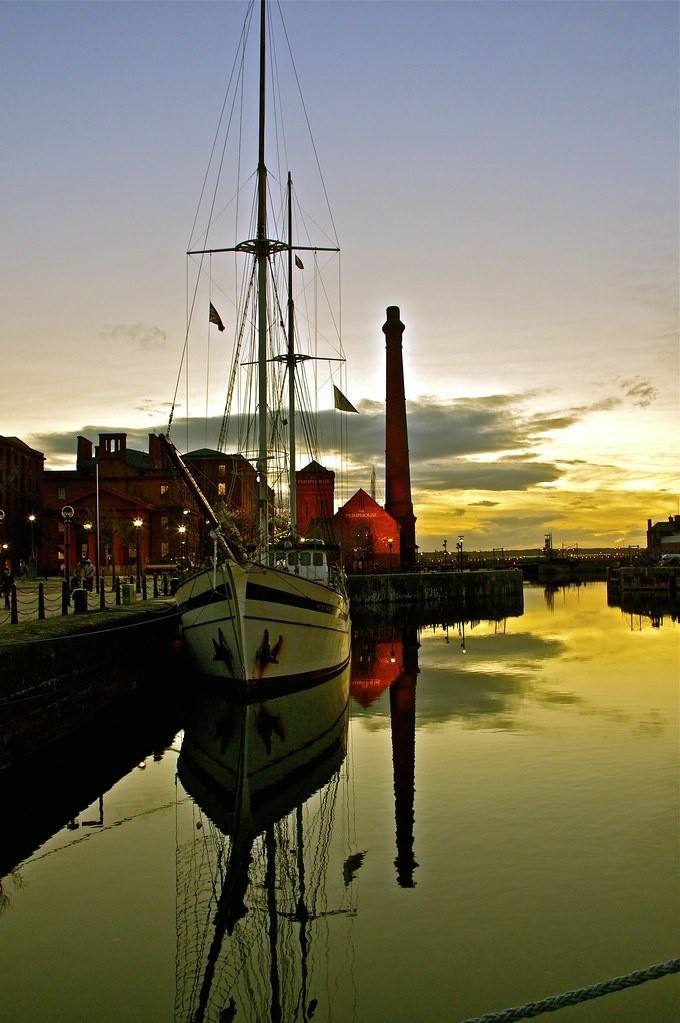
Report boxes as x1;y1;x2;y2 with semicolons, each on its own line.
70;576;86;598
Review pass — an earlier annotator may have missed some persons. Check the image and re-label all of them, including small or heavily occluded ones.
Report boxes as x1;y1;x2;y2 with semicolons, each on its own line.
1;568;14;611
20;561;29;582
85;558;95;592
60;562;64;577
70;571;81;594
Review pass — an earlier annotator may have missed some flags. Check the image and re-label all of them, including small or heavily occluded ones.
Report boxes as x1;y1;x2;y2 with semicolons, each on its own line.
208;302;225;333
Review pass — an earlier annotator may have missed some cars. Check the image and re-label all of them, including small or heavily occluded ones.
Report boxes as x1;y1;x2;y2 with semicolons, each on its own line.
150;552;176;565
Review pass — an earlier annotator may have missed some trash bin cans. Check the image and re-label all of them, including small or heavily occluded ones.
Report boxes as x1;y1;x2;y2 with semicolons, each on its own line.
170;578;179;591
73;588;87;612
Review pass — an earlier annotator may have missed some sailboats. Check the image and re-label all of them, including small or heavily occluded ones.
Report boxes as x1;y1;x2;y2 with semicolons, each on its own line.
176;659;352;1023
157;0;354;696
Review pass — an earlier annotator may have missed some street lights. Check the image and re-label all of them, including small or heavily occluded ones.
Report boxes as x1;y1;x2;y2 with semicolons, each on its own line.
133;517;143;593
83;520;93;559
2;543;7;585
444;540;447;567
179;527;187;573
460;538;463;572
389;538;393;574
62;506;74;615
29;515;36;578
